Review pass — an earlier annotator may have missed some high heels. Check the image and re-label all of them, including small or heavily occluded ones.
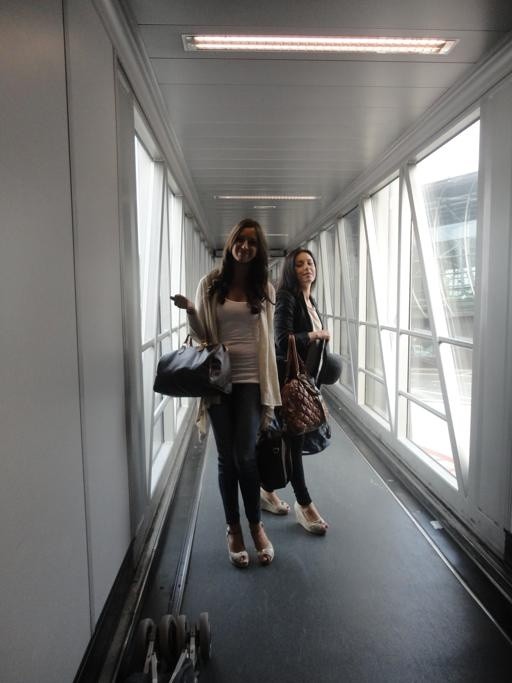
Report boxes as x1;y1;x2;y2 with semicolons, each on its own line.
294;500;327;535
259;486;290;515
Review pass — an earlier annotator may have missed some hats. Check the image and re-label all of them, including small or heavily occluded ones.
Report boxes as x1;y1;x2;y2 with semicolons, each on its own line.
305;337;342;390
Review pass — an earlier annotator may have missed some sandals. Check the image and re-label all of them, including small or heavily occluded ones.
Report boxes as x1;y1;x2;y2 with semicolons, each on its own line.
226;526;249;568
248;521;275;563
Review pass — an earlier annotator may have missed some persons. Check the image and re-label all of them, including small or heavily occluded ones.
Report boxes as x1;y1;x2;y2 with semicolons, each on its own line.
169;218;282;568
260;248;330;535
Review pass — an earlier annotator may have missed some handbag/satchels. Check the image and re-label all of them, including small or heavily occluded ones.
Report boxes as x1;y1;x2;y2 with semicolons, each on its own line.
276;334;330;437
255;430;294;492
301;421;331;455
153;327;233;397
